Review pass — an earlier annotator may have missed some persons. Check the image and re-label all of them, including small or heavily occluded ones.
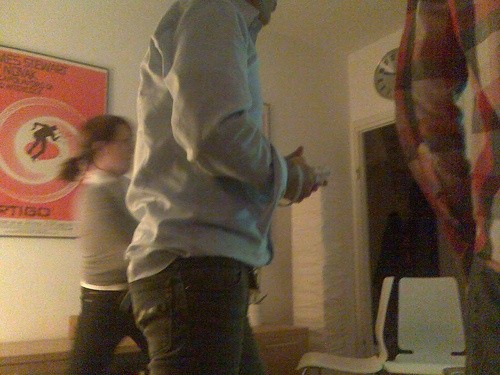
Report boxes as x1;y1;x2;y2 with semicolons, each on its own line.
61;115;153;374
124;1;329;375
393;1;500;375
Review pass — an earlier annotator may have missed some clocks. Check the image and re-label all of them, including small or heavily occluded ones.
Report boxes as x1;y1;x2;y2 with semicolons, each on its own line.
373;46;399;100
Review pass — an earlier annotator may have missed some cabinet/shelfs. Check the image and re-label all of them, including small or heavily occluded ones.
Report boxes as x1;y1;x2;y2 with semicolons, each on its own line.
1;322;309;375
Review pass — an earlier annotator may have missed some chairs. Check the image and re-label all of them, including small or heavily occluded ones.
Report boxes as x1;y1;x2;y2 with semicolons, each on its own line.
293;275;395;375
383;275;469;375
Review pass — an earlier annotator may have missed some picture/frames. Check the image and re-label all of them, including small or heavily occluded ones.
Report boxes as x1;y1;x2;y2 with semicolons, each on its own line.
0;44;109;239
